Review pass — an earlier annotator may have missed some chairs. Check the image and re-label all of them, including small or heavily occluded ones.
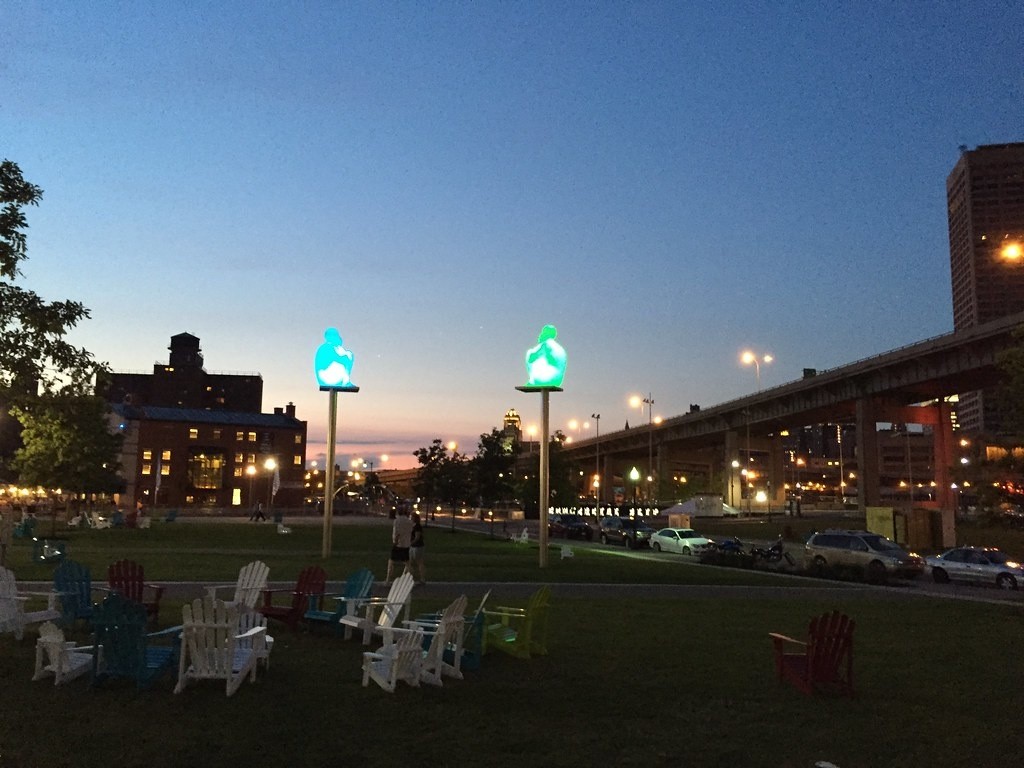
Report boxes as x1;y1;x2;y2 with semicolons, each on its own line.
171;596;268;699
360;629;424;693
204;560;270;617
415;588;491;670
0;514;68;581
225;612;274;669
560;544;574;560
32;621;103;688
67;508;151;532
510;527;529;545
768;609;857;703
335;571;416;647
160;509;177;525
384;594;468;688
0;566;63;642
107;560;167;627
258;565;327;633
473;585;552;662
304;567;375;637
91;592;179;693
274;511;292;536
54;559;113;633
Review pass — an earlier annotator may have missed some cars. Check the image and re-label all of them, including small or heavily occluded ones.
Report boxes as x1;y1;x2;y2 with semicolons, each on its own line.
923;545;1024;592
547;513;594;541
649;527;716;557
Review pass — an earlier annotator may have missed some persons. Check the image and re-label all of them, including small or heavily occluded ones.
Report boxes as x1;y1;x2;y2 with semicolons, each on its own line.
249;498;266;522
386;505;424;587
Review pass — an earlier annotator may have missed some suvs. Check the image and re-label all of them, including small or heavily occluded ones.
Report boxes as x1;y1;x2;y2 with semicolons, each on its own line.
804;528;922;586
599;514;658;549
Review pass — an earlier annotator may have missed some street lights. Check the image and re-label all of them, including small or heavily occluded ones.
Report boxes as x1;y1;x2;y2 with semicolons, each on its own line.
514;325;568;569
590;412;601;522
643;391;654;501
631;466;640;542
743;350;761;396
313;326;361;560
729;460;739;510
246;465;257;515
526;424;536;454
264;459;275;513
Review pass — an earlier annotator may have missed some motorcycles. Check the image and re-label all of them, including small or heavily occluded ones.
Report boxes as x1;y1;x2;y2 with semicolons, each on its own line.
707;536;747;560
751;534;796;567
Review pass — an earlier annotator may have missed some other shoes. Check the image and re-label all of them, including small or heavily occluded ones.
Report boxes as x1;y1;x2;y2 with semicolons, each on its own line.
420;577;426;584
384;582;391;588
